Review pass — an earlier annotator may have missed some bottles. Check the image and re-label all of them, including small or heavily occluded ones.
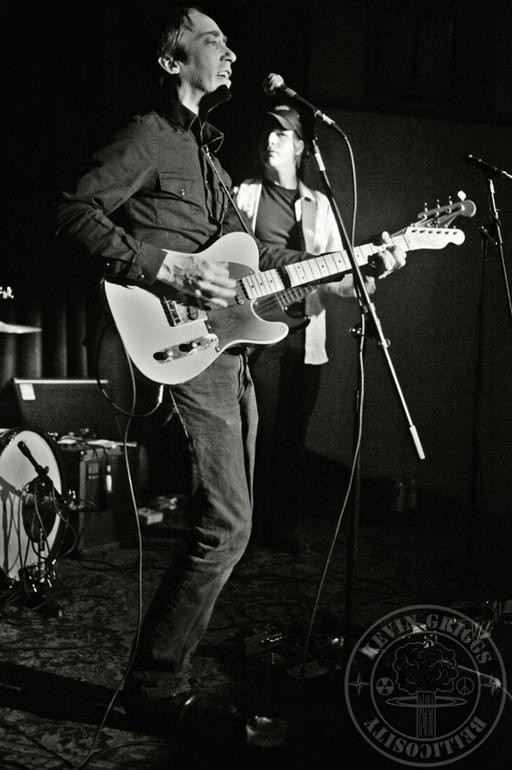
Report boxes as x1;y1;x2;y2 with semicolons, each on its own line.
390;475;418;514
244;713;290;770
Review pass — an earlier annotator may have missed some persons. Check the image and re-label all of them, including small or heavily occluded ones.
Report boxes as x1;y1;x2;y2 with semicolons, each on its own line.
233;101;394;531
48;5;405;741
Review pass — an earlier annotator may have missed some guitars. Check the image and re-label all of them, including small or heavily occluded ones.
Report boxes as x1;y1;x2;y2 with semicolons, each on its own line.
222;190;476;355
104;224;464;386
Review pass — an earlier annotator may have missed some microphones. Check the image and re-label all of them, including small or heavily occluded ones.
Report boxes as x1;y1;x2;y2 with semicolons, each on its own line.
262;72;335;128
463;153;503;175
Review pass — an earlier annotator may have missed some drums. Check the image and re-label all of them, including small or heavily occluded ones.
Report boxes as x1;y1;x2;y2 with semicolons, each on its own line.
0;428;63;580
